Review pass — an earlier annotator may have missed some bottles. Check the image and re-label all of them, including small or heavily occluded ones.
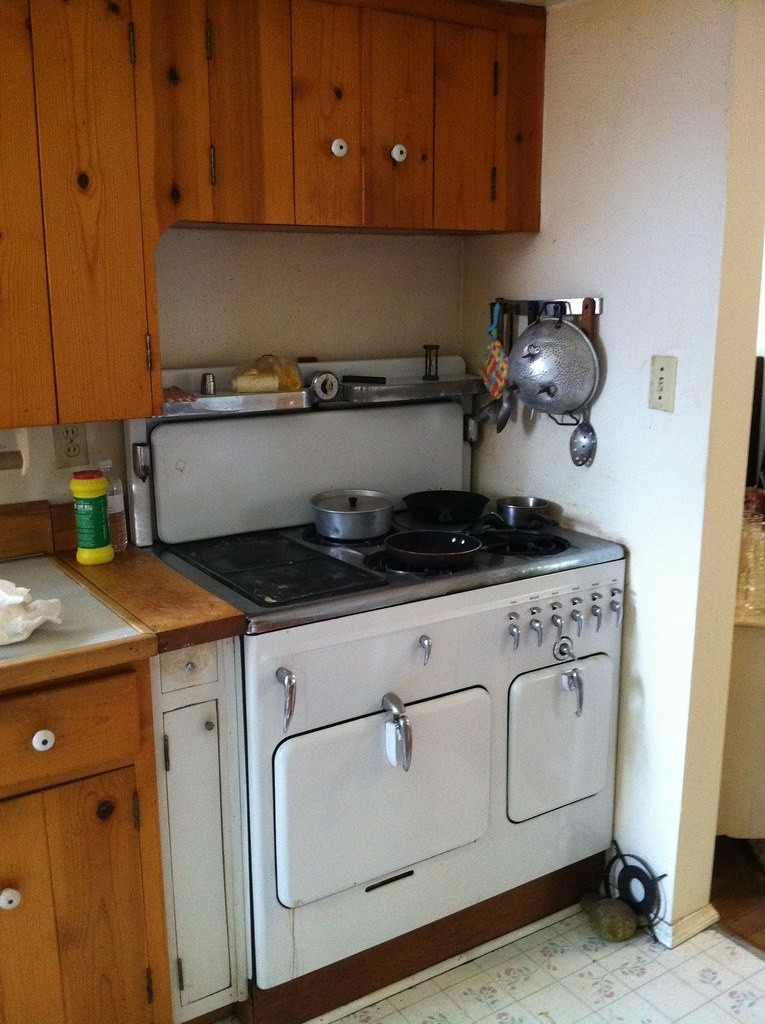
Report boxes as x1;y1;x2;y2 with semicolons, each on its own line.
98;460;127;552
70;470;114;566
201;373;215;395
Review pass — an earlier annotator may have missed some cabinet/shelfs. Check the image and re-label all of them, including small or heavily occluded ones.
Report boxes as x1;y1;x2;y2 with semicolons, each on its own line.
0;636;173;1024
165;0;547;233
150;634;247;1024
0;0;167;429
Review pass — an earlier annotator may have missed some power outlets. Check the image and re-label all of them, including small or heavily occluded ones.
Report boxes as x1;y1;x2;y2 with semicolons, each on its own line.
648;355;678;413
52;423;90;470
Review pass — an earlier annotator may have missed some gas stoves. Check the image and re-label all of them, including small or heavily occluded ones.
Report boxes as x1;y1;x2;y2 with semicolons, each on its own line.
148;404;625;636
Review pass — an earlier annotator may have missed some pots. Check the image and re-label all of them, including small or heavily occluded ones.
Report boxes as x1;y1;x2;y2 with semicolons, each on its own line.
310;488;396;540
506;301;599;425
383;490;551;572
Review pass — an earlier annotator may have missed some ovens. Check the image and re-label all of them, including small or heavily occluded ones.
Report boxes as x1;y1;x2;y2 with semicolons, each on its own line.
242;554;628;988
